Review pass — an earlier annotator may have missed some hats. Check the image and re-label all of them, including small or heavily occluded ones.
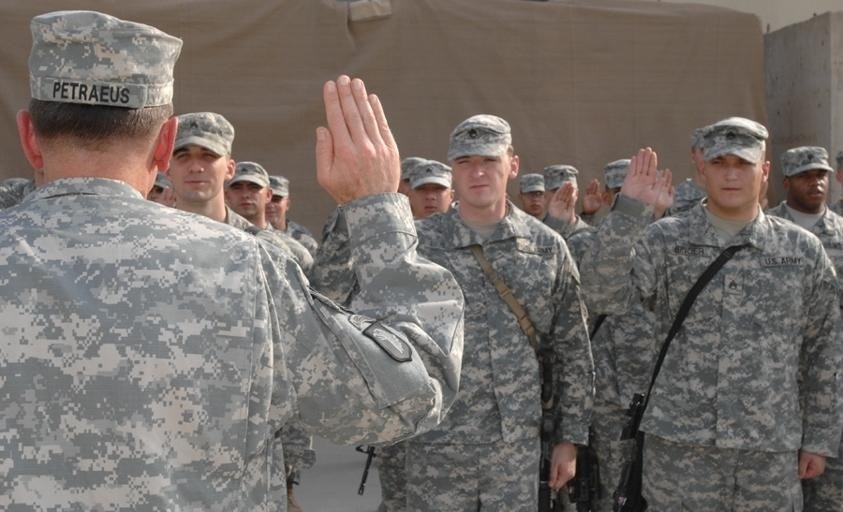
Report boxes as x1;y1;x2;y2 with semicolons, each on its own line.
1;178;29;208
152;112;289;200
401;157;453;194
518;164;579;193
691;116;843;176
603;160;631;189
446;113;512;161
28;10;183;108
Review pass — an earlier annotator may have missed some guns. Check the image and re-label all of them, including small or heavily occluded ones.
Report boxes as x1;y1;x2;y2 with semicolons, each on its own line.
612;393;649;512
354;443;378;494
538;381;558;511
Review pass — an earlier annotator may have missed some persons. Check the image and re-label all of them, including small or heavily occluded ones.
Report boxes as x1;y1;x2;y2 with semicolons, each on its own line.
1;10;466;511
306;115;843;510
